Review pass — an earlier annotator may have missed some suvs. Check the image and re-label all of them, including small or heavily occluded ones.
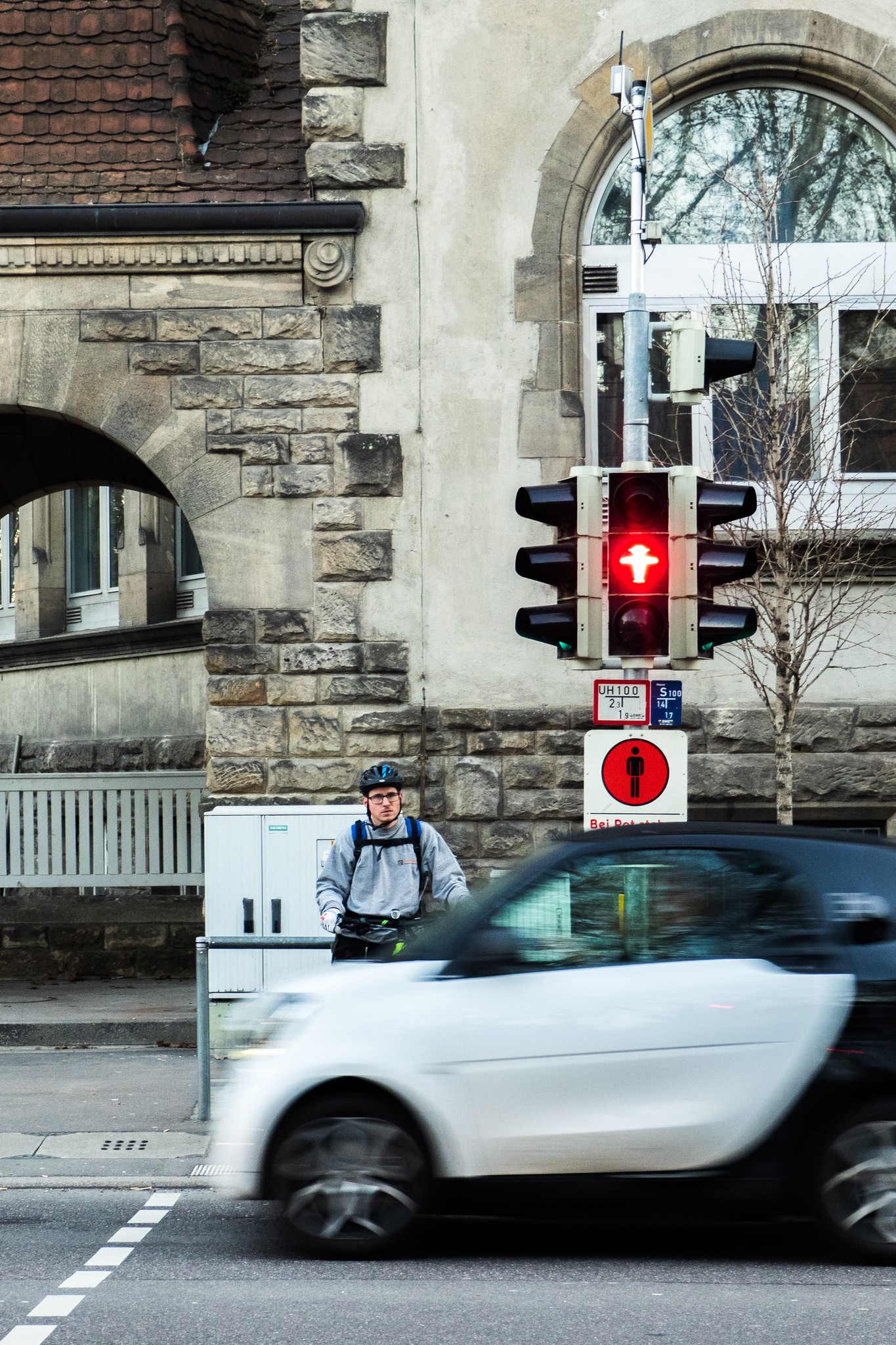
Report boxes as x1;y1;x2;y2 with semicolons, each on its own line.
202;824;896;1263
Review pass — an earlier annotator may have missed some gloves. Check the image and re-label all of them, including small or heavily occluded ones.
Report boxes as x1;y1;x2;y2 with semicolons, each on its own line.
320;908;342;934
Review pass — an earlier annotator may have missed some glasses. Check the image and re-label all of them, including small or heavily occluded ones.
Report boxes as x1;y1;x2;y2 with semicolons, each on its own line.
369;792;400;804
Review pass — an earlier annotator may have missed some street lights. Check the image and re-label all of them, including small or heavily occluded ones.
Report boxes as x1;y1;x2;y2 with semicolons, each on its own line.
608;27;658;736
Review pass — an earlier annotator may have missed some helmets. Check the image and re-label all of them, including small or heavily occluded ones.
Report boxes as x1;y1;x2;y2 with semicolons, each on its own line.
359;765;403;793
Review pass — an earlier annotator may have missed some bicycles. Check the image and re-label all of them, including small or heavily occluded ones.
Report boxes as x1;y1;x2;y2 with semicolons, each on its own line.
333;915;453;965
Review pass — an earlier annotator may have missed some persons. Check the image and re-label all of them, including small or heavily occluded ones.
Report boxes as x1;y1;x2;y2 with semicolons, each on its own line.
316;765;471;964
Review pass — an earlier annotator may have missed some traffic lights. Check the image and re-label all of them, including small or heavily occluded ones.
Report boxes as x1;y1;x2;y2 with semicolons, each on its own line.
611;467;670;657
666;464;759;664
675;327;760;395
513;466;604;662
